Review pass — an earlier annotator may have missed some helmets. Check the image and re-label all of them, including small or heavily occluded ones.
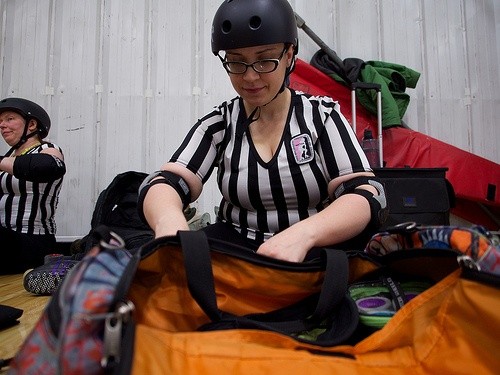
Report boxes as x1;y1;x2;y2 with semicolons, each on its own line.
211;0;298;55
0;98;50;139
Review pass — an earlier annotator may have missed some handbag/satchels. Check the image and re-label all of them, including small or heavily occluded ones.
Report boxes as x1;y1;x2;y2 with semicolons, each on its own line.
1;220;500;375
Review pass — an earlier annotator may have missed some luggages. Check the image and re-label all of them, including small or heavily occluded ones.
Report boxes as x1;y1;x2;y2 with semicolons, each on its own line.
350;82;456;242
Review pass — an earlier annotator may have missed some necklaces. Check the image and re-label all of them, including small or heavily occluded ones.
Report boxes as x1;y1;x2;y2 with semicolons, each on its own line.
20;142;42;156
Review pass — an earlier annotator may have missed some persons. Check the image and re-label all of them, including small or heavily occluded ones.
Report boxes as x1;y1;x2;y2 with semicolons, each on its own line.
23;1;387;298
0;98;67;275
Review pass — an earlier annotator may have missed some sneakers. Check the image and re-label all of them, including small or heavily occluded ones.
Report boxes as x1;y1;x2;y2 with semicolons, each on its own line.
22;259;81;295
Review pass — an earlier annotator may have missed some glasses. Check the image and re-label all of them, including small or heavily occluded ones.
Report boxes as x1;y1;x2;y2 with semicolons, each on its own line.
222;42;287;74
